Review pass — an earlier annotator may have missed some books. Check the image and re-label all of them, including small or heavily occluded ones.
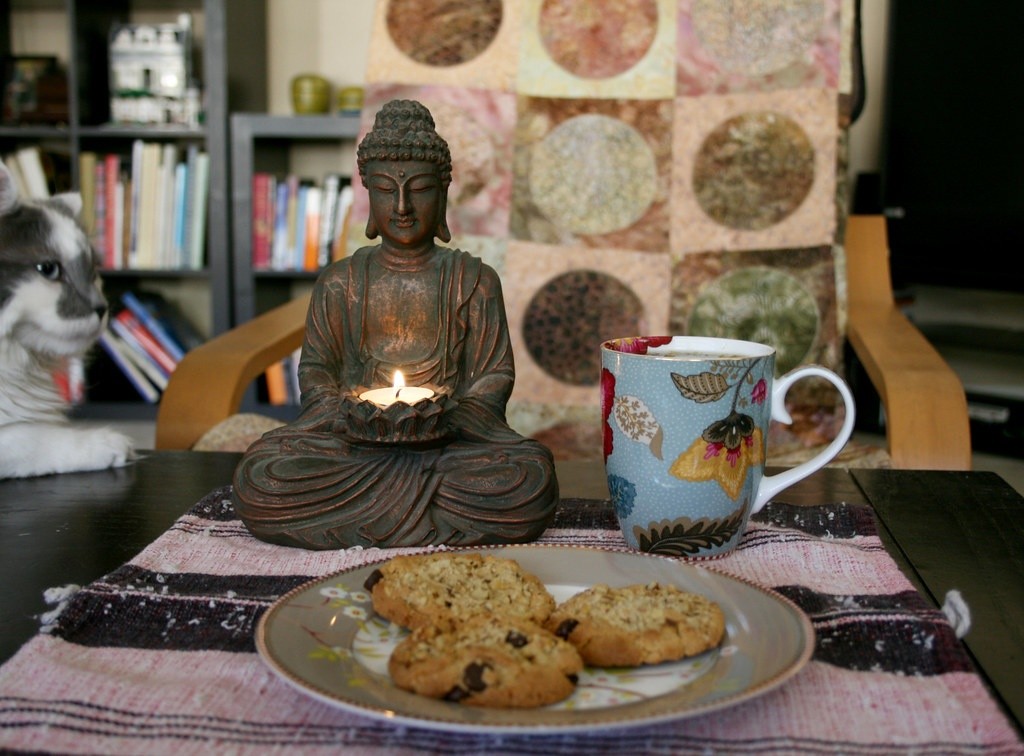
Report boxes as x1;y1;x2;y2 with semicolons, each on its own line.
250;169;354;405
5;138;210;404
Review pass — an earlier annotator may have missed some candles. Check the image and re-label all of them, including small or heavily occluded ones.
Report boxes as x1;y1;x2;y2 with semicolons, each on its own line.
360;369;434;404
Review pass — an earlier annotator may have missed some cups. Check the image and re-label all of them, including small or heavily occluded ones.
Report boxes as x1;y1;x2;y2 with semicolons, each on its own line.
292;75;331;116
600;333;856;557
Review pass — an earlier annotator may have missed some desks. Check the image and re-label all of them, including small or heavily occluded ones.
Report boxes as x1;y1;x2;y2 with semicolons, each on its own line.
0;448;1024;756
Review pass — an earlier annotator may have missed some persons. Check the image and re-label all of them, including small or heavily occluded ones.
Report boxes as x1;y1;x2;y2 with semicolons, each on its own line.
231;99;559;551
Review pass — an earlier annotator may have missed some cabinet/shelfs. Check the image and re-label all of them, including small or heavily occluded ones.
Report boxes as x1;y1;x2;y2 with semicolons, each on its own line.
0;0;362;425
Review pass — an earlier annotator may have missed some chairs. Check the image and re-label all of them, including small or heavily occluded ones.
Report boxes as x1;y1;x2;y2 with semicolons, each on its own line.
161;0;971;471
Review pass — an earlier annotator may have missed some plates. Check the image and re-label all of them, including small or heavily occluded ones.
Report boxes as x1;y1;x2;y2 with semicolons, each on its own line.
255;544;817;735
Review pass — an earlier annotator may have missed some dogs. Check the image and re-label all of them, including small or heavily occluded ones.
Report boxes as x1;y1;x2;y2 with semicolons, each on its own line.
2;161;130;480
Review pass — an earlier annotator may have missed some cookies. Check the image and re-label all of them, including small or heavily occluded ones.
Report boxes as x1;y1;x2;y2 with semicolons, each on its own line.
368;550;726;709
